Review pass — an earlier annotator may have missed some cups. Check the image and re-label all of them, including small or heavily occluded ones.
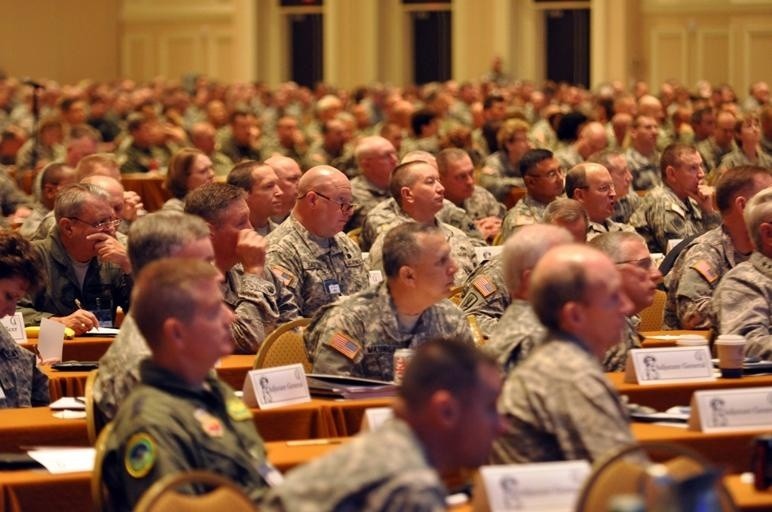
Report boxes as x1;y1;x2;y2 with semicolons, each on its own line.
715;333;748;380
673;333;710;348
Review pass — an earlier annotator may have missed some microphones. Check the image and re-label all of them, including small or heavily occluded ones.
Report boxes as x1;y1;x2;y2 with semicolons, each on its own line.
23;76;46;90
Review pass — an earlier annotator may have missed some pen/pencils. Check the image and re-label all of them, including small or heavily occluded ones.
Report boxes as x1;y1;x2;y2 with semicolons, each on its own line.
74;397;85;404
74;298;99;331
32;345;44;361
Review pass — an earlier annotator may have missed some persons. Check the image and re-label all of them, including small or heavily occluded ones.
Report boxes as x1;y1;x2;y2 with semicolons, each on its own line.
1;72;772;509
478;52;511;89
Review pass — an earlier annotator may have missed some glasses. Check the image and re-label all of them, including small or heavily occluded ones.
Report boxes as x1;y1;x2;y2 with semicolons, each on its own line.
572;182;616;195
60;215;122;234
615;257;657;271
297;189;356;216
524;166;568;181
